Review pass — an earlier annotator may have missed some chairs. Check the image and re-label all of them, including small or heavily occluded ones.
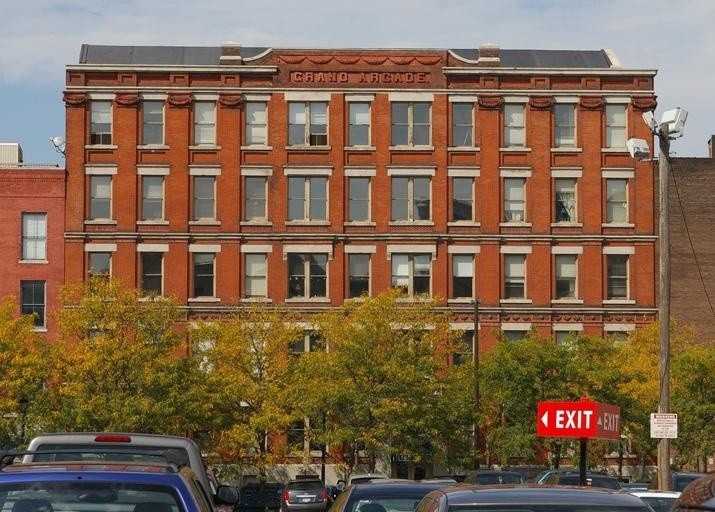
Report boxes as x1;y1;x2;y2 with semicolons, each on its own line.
360;503;386;511
11;499;52;511
134;501;173;512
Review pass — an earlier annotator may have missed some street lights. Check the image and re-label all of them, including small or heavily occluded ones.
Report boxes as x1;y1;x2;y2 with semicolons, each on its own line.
626;108;690;491
470;297;478;469
